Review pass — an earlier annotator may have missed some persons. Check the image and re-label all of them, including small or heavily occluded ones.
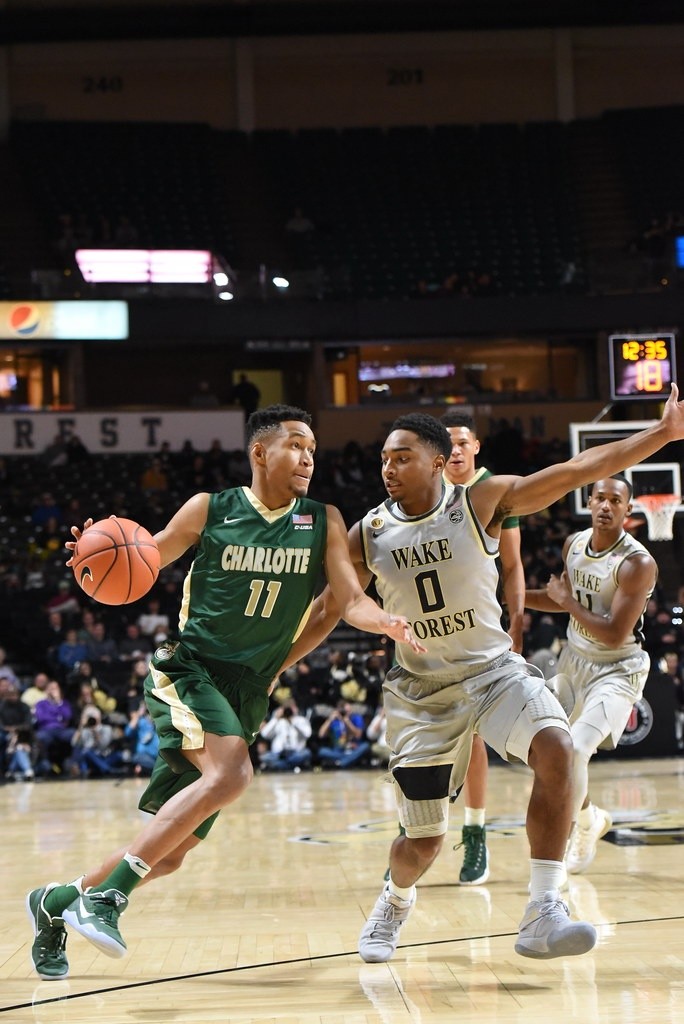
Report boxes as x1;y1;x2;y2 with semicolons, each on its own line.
0;369;684;891
26;406;427;981
263;382;684;964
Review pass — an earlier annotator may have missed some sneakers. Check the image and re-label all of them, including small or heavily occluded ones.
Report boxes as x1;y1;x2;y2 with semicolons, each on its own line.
564;805;611;874
359;883;416;964
514;890;598;959
62;885;131;959
453;825;490;886
26;882;70;980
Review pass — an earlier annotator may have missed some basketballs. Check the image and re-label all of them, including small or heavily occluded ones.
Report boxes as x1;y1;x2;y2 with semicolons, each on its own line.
72;518;161;605
